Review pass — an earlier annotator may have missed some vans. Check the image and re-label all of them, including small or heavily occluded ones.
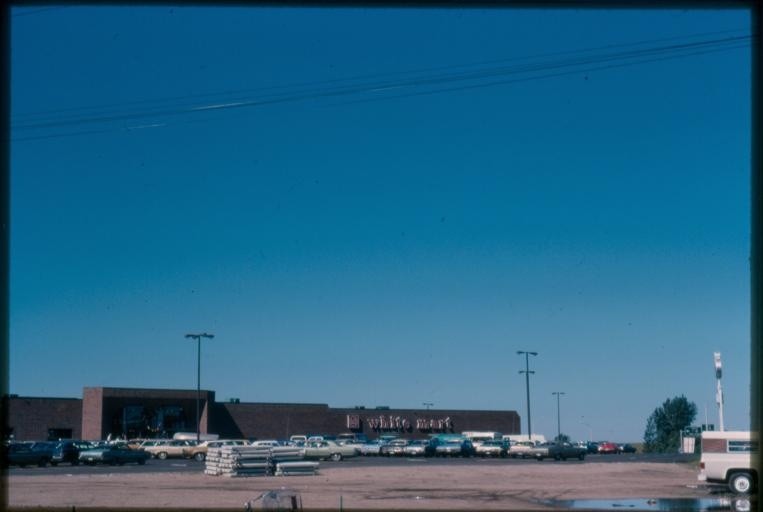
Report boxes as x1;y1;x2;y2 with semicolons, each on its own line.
720;498;751;512
697;431;760;495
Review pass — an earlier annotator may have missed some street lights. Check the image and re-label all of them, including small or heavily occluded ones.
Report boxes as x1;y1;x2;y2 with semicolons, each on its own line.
185;332;214;445
517;351;537;440
552;392;564;440
